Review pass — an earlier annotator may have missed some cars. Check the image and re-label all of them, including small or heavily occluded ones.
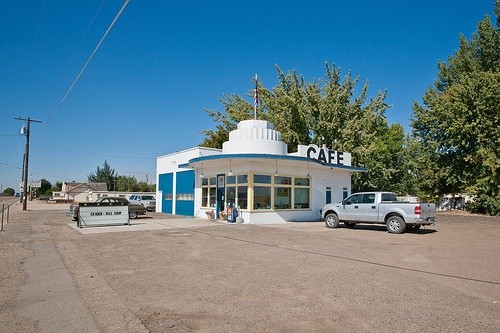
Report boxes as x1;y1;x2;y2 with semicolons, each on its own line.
15;193;20;197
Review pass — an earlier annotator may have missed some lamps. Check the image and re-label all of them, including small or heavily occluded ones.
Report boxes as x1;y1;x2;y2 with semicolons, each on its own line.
274;160;279;177
200;162;204;177
228;159;232;176
306;162;310;178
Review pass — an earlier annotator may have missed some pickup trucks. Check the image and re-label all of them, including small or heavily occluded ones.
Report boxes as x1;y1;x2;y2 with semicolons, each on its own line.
322;192;437;233
66;197;145;220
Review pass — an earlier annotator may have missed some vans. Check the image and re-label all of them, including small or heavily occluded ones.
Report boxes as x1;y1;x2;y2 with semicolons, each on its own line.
129;194;156;211
